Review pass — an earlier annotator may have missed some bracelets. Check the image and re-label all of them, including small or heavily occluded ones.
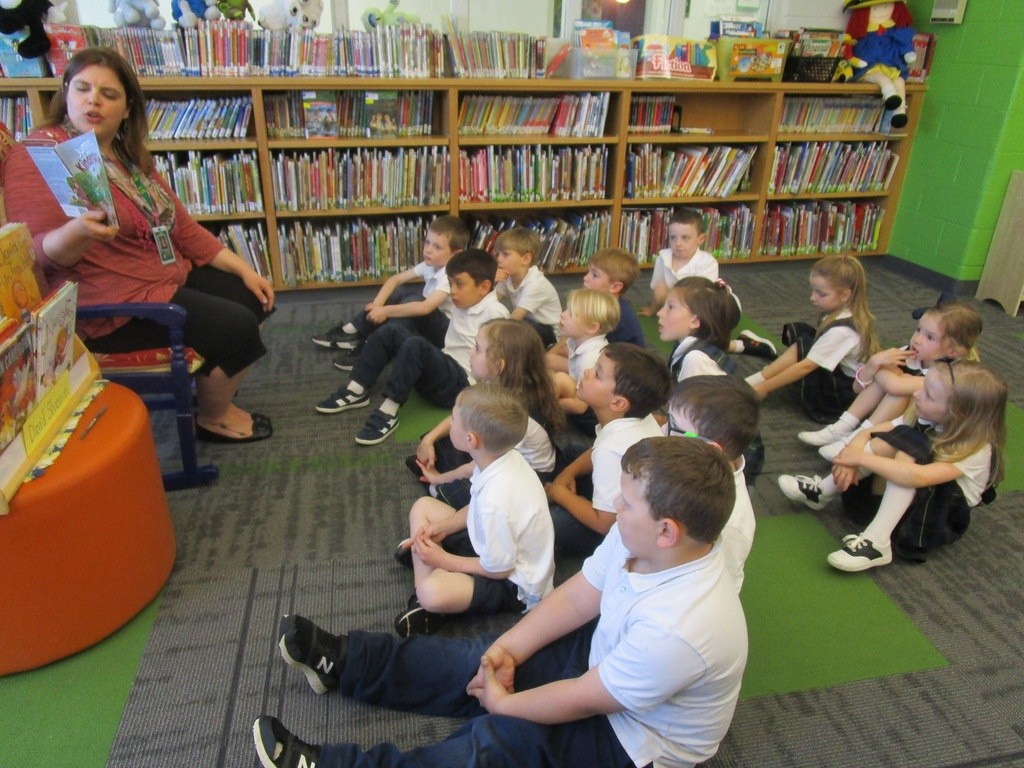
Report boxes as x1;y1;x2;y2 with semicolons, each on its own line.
855;367;874;388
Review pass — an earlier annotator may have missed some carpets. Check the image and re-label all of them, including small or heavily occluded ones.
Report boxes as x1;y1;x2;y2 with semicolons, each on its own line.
736;510;950;701
0;596;162;768
384;353;453;442
633;303;787;354
868;401;1024;492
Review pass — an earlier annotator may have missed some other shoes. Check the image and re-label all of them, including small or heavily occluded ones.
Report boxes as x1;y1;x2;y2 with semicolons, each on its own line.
798;425;853;446
818;440;850;461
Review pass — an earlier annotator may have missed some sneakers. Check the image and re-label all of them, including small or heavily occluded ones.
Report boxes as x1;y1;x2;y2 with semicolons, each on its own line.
333;344;368;371
315;385;370;413
394;595;459;638
312;321;361;349
278;614;346;695
827;532;893;572
394;538;413;567
779;474;834;511
252;716;319;768
737;330;778;359
355;405;400;445
407;454;431;488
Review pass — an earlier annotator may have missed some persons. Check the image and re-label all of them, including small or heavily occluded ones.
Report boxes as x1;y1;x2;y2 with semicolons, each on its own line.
636;209;778;361
546;249;645;439
395;318;760;638
3;46;273;444
797;291;984;462
252;437;748;768
160;237;168;249
778;360;1006;571
311;216;562;445
656;277;764;499
743;254;884;424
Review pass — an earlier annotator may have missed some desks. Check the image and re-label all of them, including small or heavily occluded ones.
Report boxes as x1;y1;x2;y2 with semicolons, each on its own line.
0;377;177;677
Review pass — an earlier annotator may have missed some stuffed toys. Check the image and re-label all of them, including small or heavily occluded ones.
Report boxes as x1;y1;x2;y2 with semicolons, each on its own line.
0;0;420;60
830;0;917;128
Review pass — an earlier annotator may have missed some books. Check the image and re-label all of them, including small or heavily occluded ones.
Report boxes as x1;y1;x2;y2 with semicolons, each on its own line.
0;221;78;455
23;128;120;231
0;19;272;282
618;15;929;265
251;13;630;284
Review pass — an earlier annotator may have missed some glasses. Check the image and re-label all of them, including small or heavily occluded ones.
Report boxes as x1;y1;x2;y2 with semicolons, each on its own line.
666;413;710;442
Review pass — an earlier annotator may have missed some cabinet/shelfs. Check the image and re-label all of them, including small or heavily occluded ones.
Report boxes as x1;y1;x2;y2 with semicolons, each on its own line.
0;20;928;290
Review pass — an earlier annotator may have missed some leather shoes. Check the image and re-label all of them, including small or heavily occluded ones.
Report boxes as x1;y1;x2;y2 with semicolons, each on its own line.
196;413;271;441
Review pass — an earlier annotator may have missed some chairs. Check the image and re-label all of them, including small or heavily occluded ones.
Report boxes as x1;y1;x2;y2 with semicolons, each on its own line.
0;123;221;491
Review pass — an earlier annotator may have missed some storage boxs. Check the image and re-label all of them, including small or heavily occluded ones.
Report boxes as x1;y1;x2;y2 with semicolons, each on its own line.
562;37;845;83
